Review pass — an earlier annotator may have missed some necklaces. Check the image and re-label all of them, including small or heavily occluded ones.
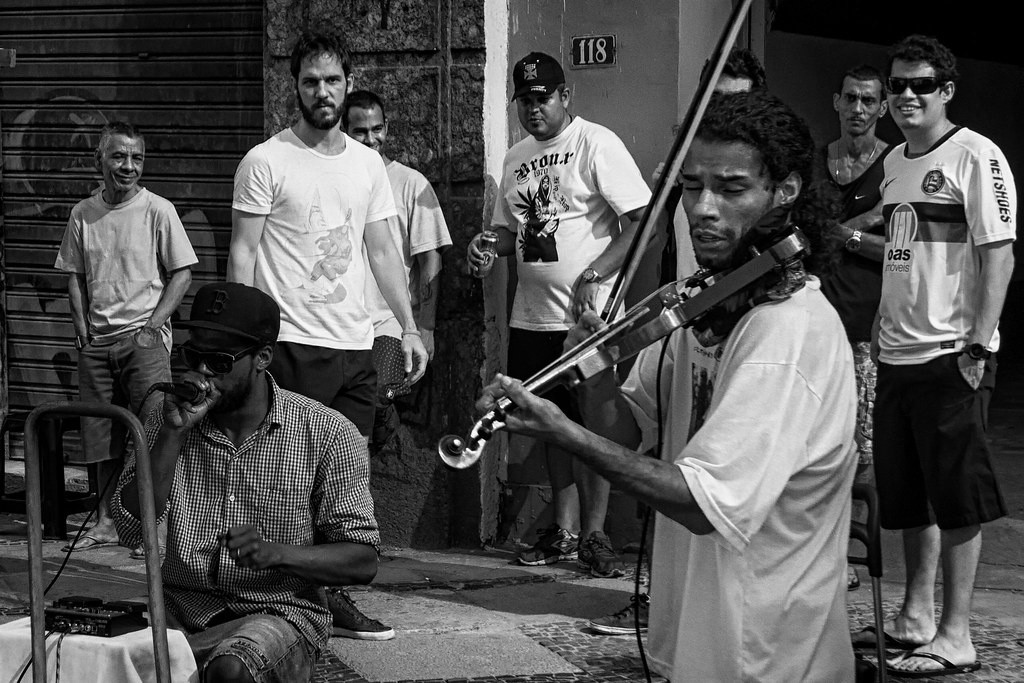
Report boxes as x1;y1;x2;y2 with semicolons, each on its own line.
568;113;572;124
834;139;880;185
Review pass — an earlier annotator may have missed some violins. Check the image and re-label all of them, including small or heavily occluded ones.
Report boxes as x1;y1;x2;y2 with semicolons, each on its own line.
437;233;812;474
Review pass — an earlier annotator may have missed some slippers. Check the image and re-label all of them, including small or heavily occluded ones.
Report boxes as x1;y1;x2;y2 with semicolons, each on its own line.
885;649;982;676
63;535;118;552
852;625;925;650
131;543;166;559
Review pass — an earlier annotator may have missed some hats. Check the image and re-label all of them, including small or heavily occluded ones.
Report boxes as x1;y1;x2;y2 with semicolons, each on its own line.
172;282;281;345
511;51;566;102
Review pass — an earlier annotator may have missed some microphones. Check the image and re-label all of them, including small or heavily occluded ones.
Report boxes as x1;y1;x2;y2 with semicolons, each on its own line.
157;382;207;406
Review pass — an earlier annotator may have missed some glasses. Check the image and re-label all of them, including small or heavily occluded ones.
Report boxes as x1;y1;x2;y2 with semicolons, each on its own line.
177;339;256;375
884;76;946;95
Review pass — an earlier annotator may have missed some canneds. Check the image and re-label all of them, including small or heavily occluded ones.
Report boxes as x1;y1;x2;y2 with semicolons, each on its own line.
472;230;499;278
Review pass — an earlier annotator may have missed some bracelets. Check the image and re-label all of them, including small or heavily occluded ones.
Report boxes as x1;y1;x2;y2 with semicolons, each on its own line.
401;330;422;340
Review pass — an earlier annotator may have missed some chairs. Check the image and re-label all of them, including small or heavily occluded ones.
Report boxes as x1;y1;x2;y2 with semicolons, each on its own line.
845;339;891;683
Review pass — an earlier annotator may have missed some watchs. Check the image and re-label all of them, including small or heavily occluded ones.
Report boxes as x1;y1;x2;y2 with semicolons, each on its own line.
581;267;602;284
75;336;89;349
960;342;992;360
845;228;863;254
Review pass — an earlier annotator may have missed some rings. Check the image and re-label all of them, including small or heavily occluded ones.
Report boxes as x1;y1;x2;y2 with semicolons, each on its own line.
236;549;242;559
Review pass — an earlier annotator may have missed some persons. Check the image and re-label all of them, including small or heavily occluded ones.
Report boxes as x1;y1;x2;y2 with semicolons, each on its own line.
112;279;381;683
53;119;201;560
341;89;452;453
567;34;1024;682
465;52;660;581
226;26;429;642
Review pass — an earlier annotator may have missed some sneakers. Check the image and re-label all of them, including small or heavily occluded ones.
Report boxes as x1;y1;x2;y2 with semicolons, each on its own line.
589;592;651;634
576;529;625;578
324;588;395;641
517;524;579;566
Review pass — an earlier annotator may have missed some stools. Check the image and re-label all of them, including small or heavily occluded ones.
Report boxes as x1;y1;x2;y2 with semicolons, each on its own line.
0;613;200;683
